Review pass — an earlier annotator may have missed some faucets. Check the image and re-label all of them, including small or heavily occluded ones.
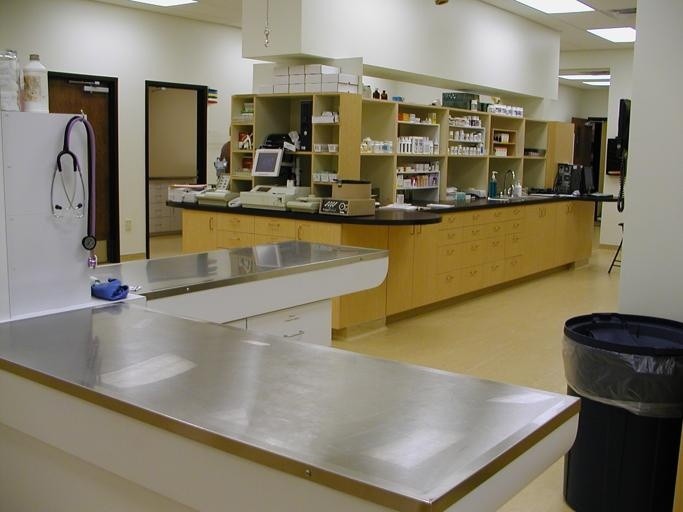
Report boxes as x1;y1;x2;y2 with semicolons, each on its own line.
503;169;516;194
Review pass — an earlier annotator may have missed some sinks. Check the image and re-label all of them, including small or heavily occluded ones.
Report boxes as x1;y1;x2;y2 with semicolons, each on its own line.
488;193;554;202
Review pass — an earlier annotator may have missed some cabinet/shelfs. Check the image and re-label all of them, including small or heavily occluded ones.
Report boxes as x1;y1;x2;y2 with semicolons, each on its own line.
181;208;387;341
147;177;197;234
365;93;546;200
386;200;595;326
230;92;363;191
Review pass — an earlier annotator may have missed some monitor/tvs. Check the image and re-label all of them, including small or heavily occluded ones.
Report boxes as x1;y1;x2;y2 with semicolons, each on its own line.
252;149;283;177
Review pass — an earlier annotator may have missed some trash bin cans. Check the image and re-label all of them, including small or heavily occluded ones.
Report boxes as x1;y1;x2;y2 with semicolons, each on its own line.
564;313;683;511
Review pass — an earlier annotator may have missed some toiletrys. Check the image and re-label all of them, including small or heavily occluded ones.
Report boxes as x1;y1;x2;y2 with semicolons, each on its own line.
489;170;499;197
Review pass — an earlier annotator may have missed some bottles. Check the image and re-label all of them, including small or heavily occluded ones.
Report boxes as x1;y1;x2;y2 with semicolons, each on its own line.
373;89;388;100
486;104;523;117
471;99;477;111
448;129;484;155
0;49;50;113
369;140;392;153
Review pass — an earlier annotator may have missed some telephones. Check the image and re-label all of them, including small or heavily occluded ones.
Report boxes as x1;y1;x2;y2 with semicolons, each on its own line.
606;137;627;176
